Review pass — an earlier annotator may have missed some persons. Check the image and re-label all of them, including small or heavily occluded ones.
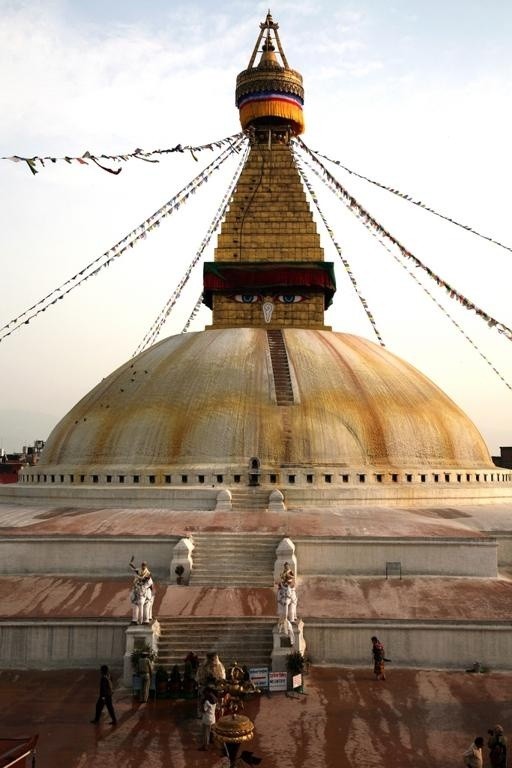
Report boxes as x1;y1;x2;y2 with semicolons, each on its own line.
278;561;296;588
197;691;219;752
137;651;254;719
370;636;387;681
89;664;118;726
129;555;156;596
463;737;486;768
488;724;508;768
486;728;495;750
